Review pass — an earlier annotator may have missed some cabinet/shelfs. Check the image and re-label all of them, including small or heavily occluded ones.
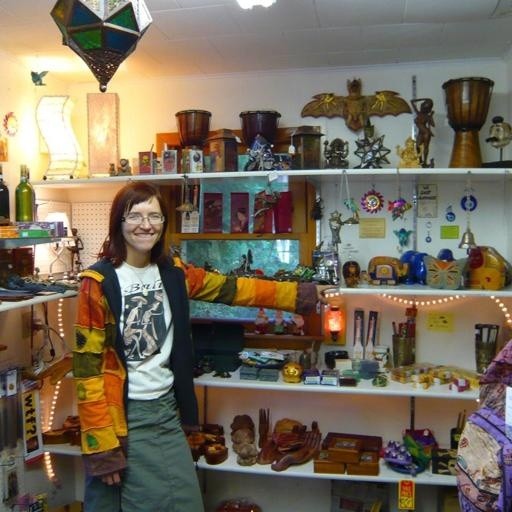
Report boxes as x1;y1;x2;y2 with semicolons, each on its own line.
0;168;511;512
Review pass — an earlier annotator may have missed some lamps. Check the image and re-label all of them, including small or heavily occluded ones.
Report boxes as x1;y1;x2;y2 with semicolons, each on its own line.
50;0;153;93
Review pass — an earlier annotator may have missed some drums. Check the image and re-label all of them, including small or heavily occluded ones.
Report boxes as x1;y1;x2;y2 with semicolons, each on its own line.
176;109;211;150
240;111;281;171
443;77;495;168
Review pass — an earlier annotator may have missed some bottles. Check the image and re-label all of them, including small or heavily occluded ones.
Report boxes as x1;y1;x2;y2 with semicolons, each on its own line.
0;163;36;225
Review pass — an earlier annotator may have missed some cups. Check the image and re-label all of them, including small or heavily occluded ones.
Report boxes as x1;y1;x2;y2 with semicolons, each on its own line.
361;360;377;379
393;334;412;368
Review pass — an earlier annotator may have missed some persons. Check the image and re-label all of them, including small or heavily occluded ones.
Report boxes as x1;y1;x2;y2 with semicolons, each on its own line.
73;181;339;511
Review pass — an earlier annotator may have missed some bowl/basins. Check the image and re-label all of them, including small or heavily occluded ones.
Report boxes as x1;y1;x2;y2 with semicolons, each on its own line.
324;350;349;370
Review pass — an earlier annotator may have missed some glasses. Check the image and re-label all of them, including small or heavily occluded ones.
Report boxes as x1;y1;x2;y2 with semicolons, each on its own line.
120;213;165;225
